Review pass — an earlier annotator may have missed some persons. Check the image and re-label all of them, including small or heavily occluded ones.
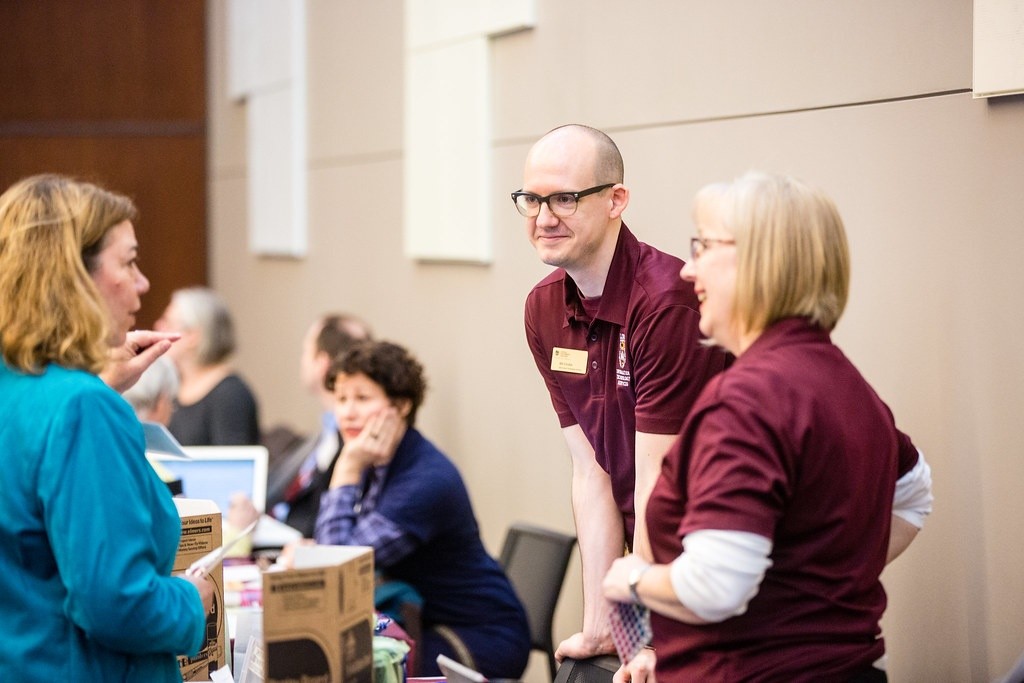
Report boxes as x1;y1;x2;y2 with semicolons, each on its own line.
511;123;735;683
120;288;261;446
0;175;216;683
604;172;935;683
266;314;533;683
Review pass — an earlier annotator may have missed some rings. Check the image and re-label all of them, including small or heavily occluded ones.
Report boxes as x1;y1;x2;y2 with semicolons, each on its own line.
369;432;380;440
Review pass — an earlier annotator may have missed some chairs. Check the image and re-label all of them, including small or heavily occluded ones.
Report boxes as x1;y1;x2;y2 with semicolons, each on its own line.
498;524;579;683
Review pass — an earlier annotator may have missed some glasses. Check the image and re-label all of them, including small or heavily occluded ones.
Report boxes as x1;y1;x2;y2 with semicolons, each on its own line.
511;183;616;218
690;238;735;259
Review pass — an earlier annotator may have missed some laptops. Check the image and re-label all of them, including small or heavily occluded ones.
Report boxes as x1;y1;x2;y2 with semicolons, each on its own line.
144;445;268;519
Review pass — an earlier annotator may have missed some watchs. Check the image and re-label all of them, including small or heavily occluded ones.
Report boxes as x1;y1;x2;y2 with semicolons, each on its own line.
626;563;652;610
